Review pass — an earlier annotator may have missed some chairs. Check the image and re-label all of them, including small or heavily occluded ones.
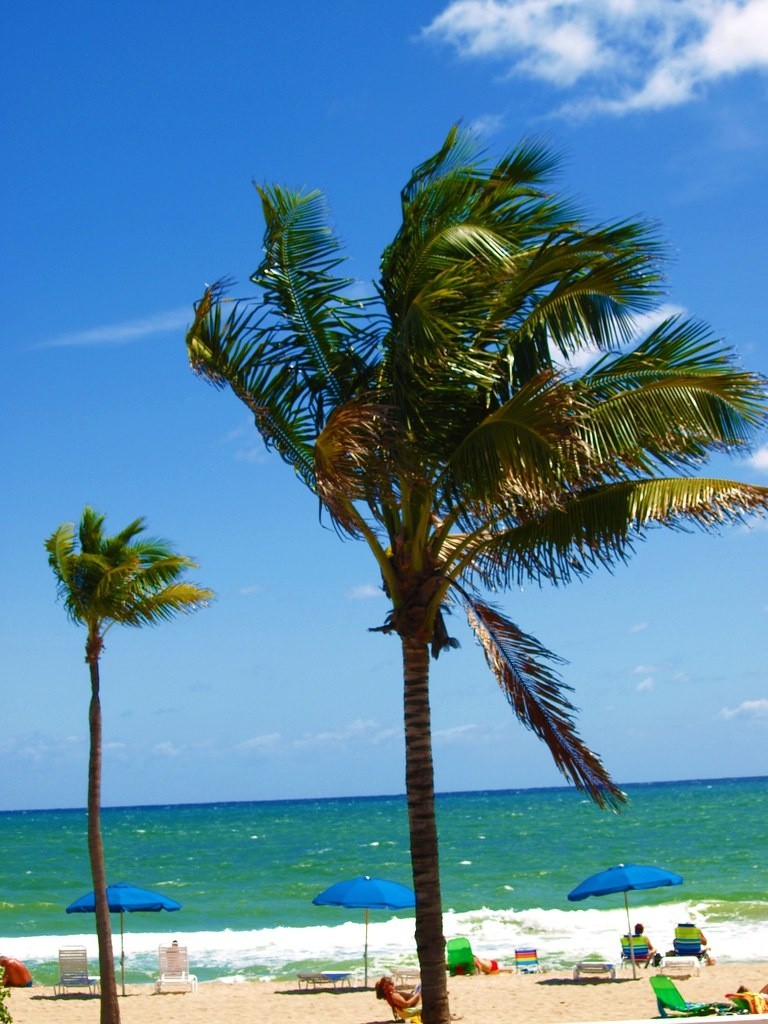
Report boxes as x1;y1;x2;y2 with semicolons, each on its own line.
658;954;700;979
650;974;737;1015
52;945;100;998
447;937;476;976
571;962;615;981
673;923;702;961
620;937;655;968
514;946;542;975
383;966;420;985
156;943;197;994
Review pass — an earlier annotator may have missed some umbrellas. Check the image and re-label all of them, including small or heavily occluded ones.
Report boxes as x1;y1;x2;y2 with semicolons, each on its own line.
66;881;182;995
567;862;684;979
312;874;415;988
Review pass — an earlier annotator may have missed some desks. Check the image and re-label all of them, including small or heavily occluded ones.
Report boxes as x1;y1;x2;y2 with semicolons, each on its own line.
298;970;355;991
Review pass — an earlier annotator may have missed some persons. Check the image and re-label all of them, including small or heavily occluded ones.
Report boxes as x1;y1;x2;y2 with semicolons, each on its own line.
738;984;768;993
620;923;653;954
685;920;715;965
375;976;449;1011
473;952;498;974
0;956;33;987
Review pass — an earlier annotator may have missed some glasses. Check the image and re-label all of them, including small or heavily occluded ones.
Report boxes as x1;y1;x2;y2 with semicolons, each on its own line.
389;983;394;986
